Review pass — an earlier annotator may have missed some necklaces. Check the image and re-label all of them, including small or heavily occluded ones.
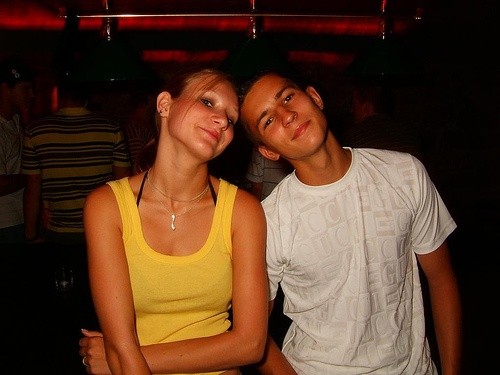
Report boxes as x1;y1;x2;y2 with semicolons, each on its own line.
147;167;210;230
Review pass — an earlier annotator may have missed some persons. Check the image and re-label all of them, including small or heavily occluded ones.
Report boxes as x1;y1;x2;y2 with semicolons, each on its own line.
1;53;28;243
21;65;132;245
18;43;472;204
78;60;269;374
230;64;463;375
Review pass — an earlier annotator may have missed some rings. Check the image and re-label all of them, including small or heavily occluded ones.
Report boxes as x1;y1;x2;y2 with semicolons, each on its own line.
82;356;88;367
